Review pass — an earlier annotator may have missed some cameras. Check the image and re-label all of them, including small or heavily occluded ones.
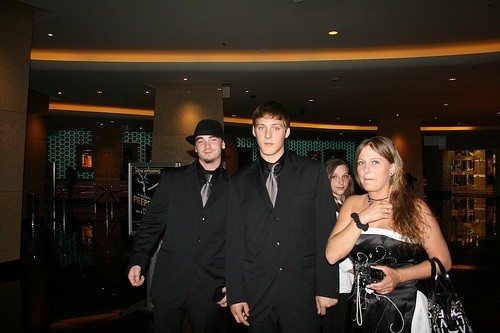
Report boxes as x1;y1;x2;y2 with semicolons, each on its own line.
367;268;385;284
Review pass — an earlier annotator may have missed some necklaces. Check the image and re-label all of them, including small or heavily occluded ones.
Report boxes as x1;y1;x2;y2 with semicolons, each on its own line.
368;195;388;202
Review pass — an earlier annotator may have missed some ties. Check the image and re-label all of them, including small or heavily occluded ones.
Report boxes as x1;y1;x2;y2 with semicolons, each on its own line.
201;174;212;208
265;163;279;208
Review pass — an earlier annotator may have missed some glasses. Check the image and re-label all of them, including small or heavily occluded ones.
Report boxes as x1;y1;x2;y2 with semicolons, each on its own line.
330;175;350;180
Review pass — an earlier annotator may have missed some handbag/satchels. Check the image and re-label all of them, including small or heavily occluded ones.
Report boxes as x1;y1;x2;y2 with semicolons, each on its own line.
428;257;472;333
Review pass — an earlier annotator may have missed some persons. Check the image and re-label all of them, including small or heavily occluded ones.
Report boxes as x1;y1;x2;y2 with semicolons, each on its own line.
226;101;338;333
128;120;233;333
324;136;452;332
324;158;355;218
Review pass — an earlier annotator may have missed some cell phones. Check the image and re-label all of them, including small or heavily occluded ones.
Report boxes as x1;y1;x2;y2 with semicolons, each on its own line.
213;291;225;302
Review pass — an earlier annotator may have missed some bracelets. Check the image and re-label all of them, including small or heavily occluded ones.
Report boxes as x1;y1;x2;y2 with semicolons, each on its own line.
351;213;367;231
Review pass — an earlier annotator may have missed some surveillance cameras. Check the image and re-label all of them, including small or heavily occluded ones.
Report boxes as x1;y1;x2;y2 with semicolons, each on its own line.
300;110;305;114
251;94;257;99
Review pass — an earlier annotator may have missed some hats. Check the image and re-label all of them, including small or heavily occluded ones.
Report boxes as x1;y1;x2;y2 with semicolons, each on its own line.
185;119;232;146
